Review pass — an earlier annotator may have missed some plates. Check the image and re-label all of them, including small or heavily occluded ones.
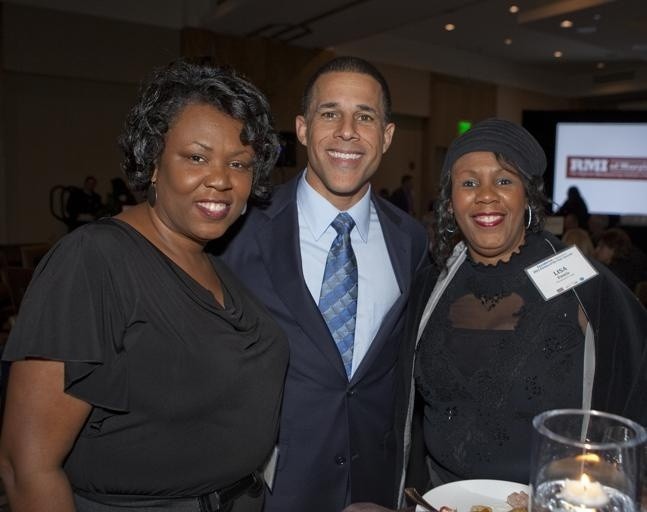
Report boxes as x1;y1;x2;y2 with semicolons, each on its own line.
414;477;530;511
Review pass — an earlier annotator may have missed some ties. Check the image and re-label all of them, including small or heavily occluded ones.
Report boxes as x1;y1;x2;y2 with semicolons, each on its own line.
318;213;358;378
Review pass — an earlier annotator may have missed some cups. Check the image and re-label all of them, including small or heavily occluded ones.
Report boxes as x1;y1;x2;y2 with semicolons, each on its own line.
531;408;646;512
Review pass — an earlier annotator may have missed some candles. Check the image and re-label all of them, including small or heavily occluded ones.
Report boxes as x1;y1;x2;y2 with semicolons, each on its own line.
558;472;609;508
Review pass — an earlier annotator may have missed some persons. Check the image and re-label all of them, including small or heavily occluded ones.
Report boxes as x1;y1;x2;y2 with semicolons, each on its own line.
210;58;438;512
554;187;645;290
380;175;438;257
413;117;646;491
0;60;287;511
66;172;138;234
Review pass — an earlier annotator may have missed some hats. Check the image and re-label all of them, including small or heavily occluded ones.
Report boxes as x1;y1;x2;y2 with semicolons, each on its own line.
445;117;548;180
